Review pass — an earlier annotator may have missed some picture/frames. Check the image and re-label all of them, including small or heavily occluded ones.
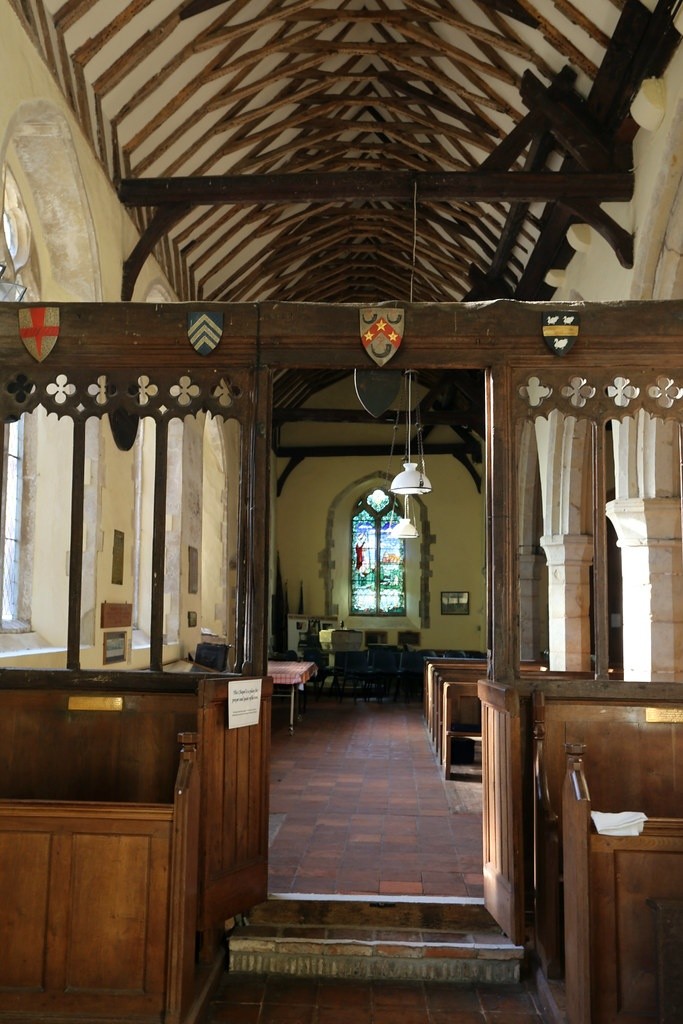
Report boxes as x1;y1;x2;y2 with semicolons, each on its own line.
441;592;469;615
103;631;126;665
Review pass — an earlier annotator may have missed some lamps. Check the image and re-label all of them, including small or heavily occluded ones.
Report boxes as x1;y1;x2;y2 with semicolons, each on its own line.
387;412;420;539
387;370;433;495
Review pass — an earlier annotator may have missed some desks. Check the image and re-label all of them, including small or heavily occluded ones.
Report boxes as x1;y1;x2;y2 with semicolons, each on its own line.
266;659;318;736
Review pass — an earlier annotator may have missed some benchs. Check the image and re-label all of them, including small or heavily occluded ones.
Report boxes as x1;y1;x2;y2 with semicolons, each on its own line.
424;657;624;780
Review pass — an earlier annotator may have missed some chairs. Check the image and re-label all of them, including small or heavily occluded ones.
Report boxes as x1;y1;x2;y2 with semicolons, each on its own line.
276;644;486;703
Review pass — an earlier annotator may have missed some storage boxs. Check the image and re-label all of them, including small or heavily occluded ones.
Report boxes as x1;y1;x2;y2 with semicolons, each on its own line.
194;642;227;672
